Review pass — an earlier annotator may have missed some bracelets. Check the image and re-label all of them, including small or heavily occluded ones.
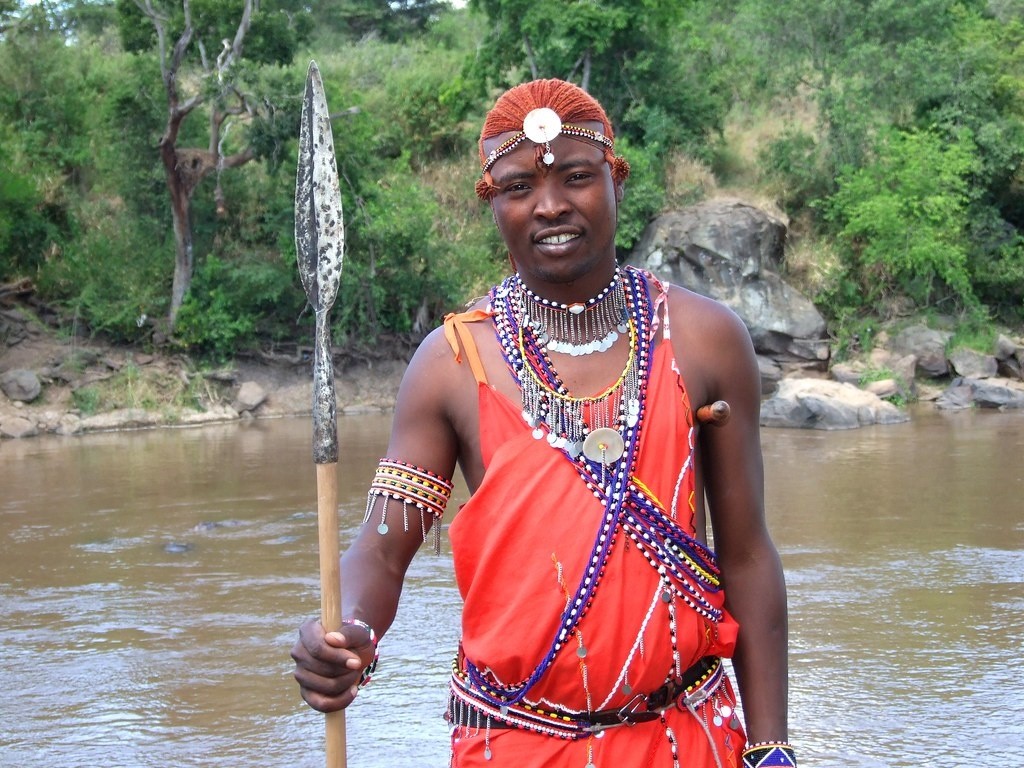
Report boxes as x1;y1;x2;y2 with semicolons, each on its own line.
343;619;377;690
742;741;797;768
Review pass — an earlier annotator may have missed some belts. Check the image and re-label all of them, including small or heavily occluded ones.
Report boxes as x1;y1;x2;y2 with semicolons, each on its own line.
449;655;718;740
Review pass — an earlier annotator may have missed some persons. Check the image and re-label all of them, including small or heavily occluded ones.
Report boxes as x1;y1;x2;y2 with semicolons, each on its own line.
290;78;798;768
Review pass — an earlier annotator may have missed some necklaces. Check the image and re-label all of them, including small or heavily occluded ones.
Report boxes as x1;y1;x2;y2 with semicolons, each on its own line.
504;257;640;439
